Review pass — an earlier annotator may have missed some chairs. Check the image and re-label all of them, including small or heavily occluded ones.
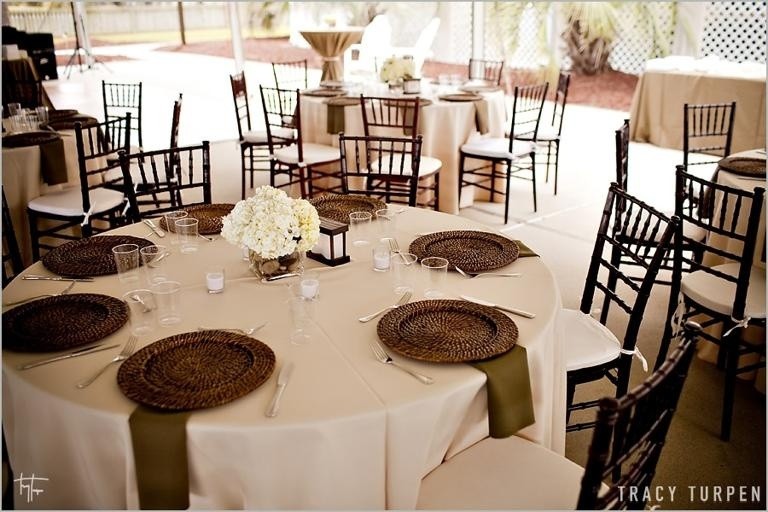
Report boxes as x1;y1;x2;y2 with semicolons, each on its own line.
599;119;706;326
663;101;736;266
562;181;681;433
229;55;571;225
2;45;212;289
652;164;767;440
415;322;703;510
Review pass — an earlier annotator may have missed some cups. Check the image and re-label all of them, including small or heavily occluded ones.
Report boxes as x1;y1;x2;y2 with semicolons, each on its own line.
7;102;48;135
350;208;449;300
288;272;321;345
422;73;462;98
112;210;225;336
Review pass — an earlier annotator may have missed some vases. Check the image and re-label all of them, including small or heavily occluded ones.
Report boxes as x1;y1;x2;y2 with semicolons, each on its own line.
248;248;303;283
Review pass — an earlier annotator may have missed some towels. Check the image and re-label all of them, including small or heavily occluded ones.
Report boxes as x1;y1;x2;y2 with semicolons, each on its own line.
512;240;540;260
465;344;535;439
128;403;193;511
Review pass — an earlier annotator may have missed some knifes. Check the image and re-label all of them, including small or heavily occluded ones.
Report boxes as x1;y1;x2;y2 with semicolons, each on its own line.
459;295;535;319
23;339;122;371
20;276;95;282
266;358;294;417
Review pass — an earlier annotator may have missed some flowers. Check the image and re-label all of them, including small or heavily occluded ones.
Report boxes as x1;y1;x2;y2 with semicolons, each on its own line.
220;185;321;259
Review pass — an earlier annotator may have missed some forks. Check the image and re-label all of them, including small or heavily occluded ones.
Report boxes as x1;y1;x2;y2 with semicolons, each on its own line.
358;290;411;322
75;336;138;389
6;280;76;308
198;322;267;336
454;265;521;280
369;340;434;385
